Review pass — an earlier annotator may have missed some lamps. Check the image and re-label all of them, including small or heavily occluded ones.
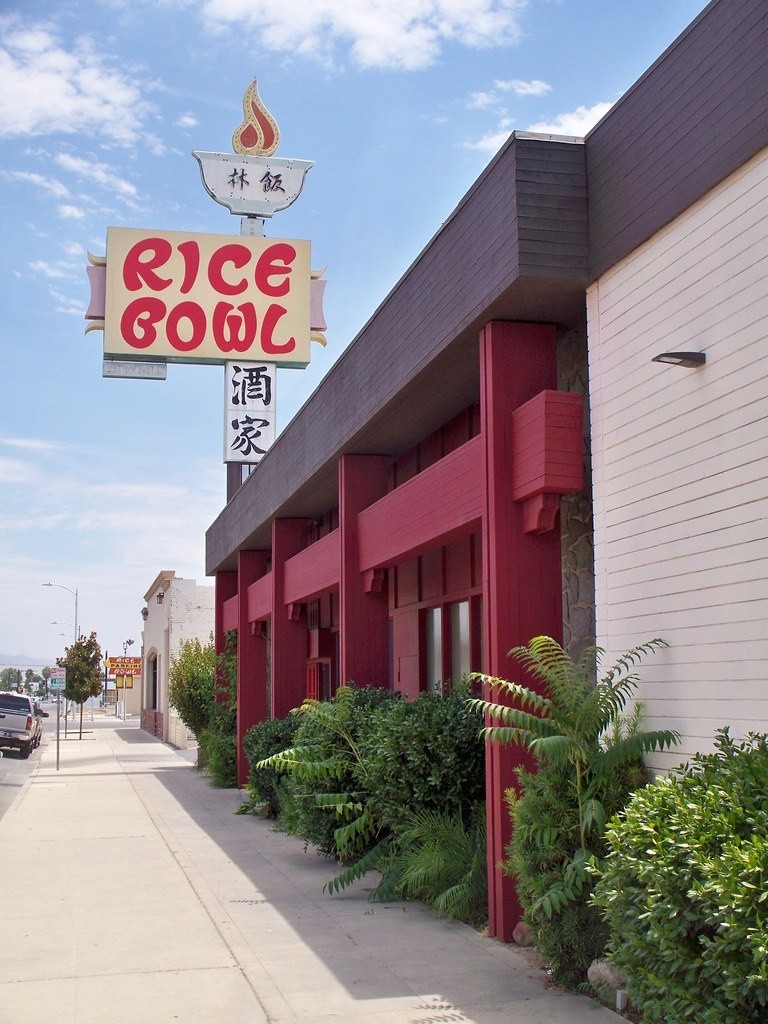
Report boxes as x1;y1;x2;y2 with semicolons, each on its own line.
652;352;705;368
143;610;148;620
156;592;164;604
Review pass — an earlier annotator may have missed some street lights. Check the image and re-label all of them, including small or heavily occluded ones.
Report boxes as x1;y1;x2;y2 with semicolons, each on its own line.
41;583;79;722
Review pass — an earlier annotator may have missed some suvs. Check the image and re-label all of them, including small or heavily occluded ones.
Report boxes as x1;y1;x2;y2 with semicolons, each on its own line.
0;691;44;760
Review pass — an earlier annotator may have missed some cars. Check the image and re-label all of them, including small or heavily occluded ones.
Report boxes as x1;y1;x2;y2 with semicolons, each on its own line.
51;695;63;703
